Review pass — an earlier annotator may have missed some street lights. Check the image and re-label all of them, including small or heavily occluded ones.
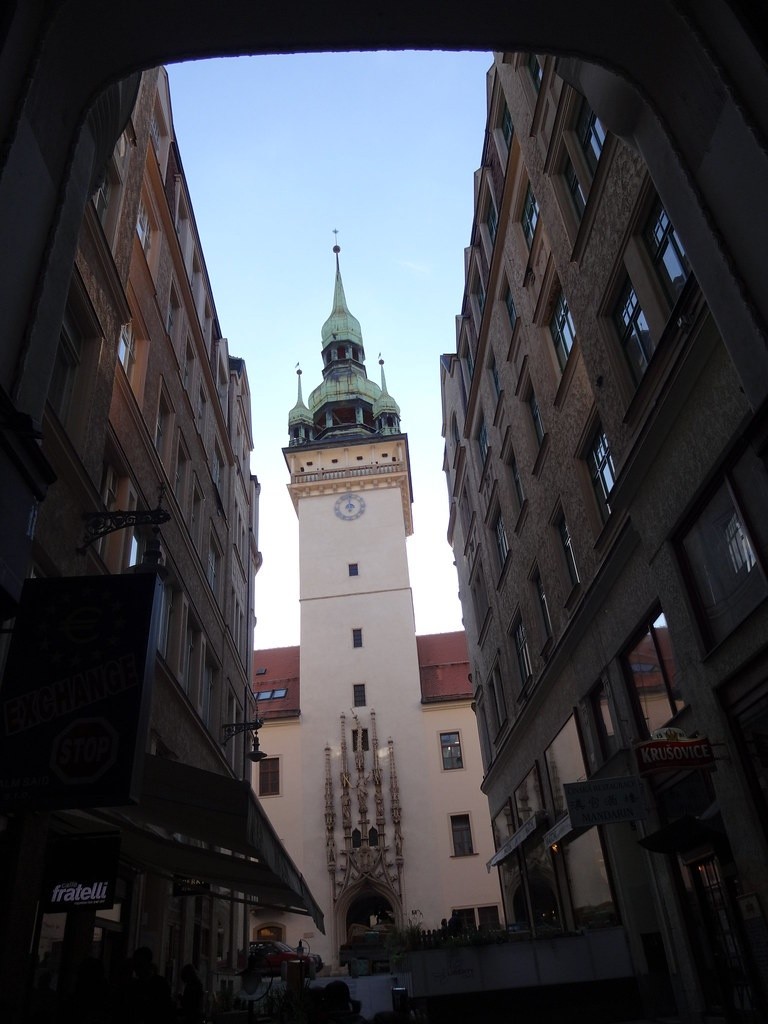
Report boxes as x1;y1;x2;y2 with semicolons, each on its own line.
296;939;305;1010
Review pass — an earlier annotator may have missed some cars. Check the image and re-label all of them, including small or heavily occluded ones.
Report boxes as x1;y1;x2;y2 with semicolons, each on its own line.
249;940;309;975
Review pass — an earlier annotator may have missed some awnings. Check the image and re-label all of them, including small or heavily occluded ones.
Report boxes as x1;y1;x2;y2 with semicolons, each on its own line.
48;747;325;933
486;811;547;873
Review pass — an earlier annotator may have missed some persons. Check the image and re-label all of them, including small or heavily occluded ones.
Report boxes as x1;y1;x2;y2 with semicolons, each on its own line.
33;937;205;1024
308;981;368;1024
